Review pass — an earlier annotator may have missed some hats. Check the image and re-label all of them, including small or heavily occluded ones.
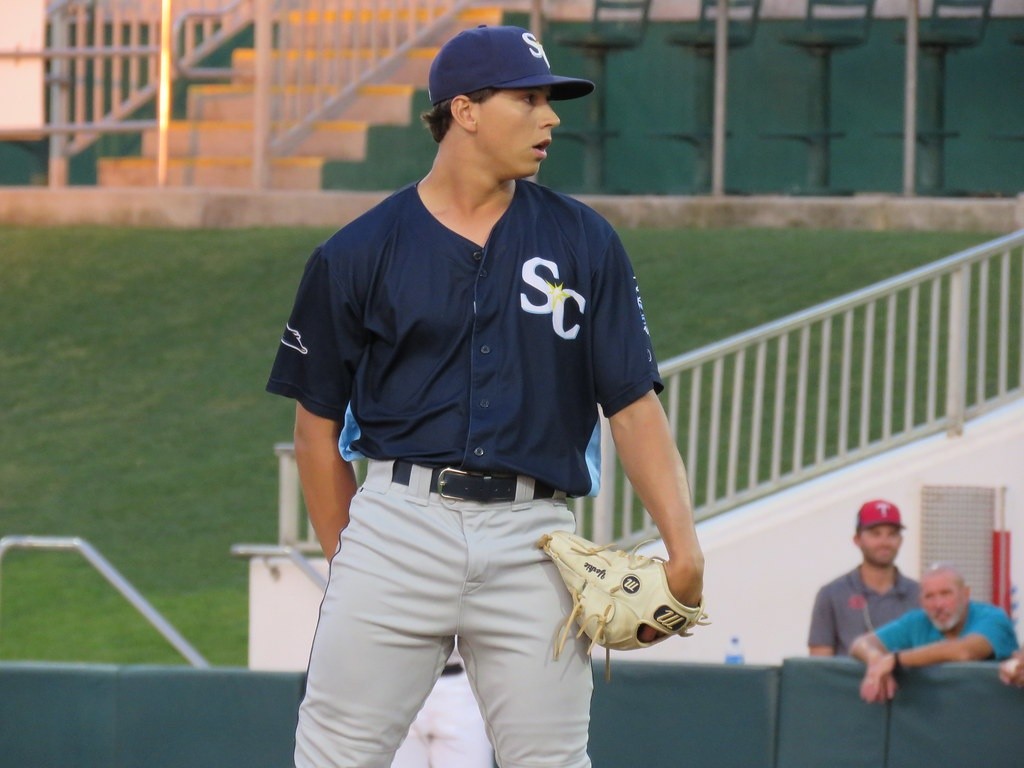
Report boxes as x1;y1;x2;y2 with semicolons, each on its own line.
428;23;595;106
856;499;906;531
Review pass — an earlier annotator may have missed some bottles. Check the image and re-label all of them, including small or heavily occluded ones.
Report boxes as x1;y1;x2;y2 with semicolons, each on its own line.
722;639;744;665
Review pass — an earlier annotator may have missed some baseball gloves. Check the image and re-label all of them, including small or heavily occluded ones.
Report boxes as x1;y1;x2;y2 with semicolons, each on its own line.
534;528;713;684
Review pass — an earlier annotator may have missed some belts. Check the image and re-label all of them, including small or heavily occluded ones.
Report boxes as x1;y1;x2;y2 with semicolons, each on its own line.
391;460;555;501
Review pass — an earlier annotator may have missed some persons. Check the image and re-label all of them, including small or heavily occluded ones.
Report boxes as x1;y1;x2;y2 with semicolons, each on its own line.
391;635;495;768
266;23;705;768
849;559;1016;700
809;501;920;656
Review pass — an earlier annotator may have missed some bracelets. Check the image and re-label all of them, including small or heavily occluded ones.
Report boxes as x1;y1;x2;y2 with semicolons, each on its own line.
894;652;900;667
